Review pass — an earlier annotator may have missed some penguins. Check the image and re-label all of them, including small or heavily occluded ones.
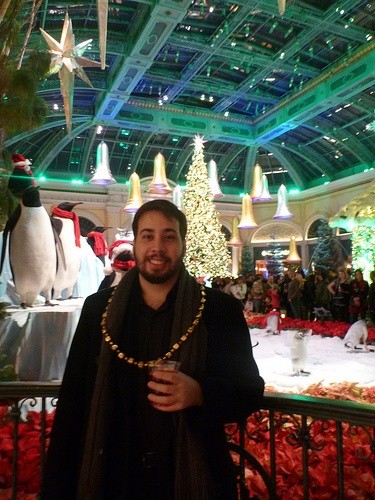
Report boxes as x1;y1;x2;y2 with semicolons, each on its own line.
50;201;114;301
288;331;311;378
0;185;57;310
261;307;284;335
342;318;370;353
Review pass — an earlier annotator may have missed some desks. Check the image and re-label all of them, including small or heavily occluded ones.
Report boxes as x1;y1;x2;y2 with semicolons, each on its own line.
0;299;85;381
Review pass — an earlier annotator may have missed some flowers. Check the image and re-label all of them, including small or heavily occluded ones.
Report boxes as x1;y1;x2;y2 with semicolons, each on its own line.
0;404;55;500
224;379;375;500
243;310;375;343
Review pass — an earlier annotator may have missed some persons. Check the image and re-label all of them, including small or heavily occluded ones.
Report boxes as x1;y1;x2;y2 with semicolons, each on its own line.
38;200;265;500
211;267;375;325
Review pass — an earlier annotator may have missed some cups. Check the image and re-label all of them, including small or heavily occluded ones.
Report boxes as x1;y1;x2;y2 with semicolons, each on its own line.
148;360;181;396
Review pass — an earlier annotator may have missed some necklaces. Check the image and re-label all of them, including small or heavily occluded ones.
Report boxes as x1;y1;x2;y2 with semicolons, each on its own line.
101;281;205;369
